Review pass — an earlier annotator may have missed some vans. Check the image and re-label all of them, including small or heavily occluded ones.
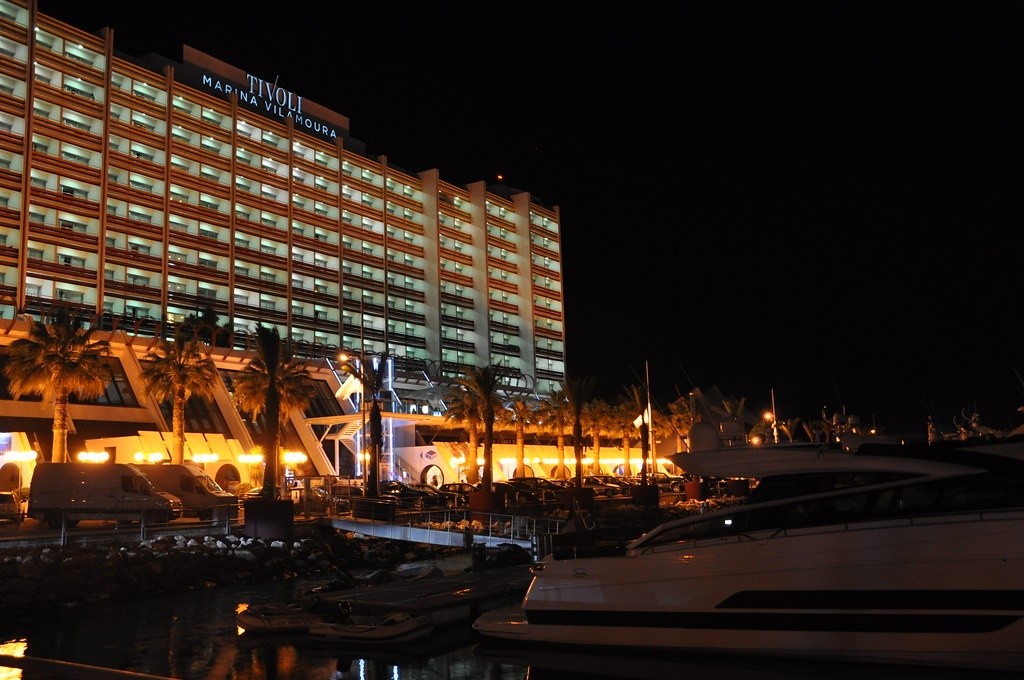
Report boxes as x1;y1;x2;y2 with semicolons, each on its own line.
135;464;238;521
27;462;184;529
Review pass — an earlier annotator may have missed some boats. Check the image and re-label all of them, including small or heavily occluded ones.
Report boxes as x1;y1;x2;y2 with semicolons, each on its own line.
232;360;1024;680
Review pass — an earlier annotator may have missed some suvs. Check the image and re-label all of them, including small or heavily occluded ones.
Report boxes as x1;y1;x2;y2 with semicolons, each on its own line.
0;491;25;523
